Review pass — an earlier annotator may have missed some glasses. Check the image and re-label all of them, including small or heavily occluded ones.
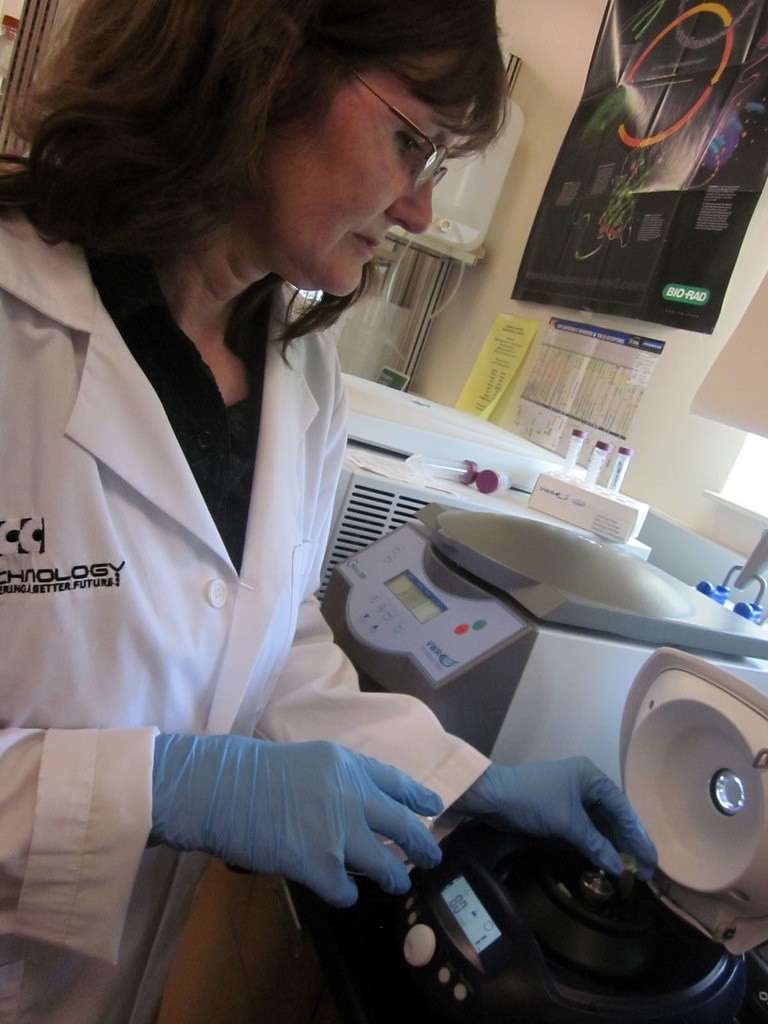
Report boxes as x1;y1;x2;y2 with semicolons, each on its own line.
344;69;447;190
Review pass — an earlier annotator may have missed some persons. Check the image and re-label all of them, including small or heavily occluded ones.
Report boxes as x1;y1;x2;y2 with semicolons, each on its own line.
0;0;660;1024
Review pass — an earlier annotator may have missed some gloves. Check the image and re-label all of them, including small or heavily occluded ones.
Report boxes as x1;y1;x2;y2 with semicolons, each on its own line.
450;754;658;881
147;733;445;909
